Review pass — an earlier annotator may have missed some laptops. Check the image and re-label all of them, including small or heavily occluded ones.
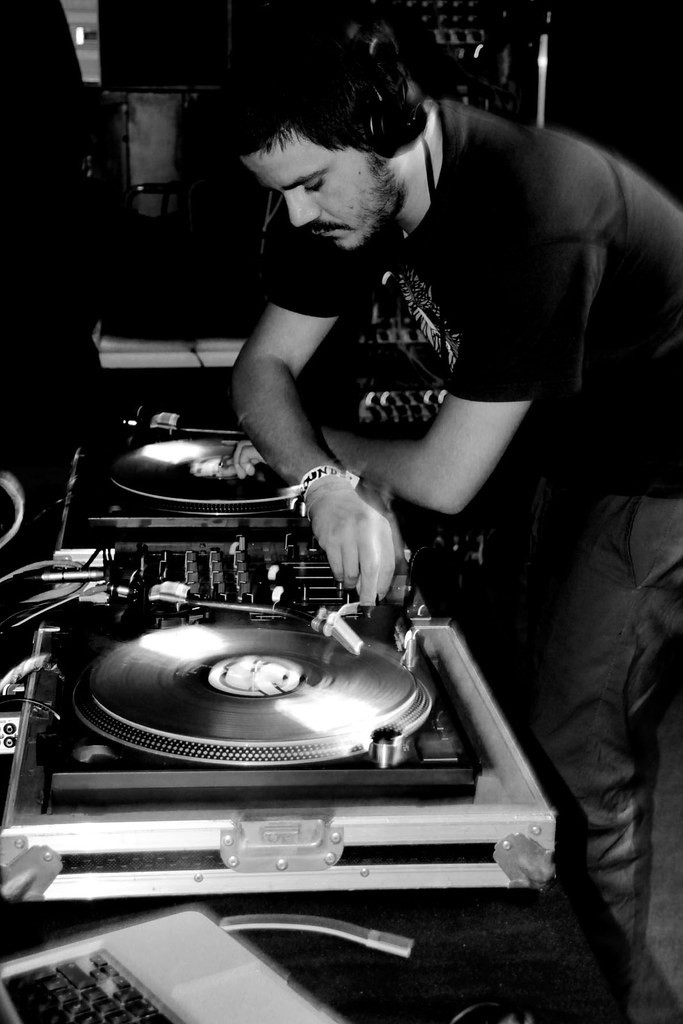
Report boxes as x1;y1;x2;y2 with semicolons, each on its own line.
0;910;335;1024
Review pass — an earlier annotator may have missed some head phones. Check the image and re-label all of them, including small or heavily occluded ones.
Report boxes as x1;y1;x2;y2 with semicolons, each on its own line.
317;20;428;158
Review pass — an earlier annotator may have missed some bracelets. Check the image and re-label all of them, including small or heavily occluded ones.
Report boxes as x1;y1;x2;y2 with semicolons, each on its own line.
298;465;344;519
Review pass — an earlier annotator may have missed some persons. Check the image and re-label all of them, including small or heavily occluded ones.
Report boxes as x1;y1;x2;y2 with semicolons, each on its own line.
227;38;683;1024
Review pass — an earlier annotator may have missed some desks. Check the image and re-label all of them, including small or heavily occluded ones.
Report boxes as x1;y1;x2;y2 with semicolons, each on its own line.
0;899;626;1024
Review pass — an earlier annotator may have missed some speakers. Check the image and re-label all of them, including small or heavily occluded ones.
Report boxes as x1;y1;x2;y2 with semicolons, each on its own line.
99;0;232;93
